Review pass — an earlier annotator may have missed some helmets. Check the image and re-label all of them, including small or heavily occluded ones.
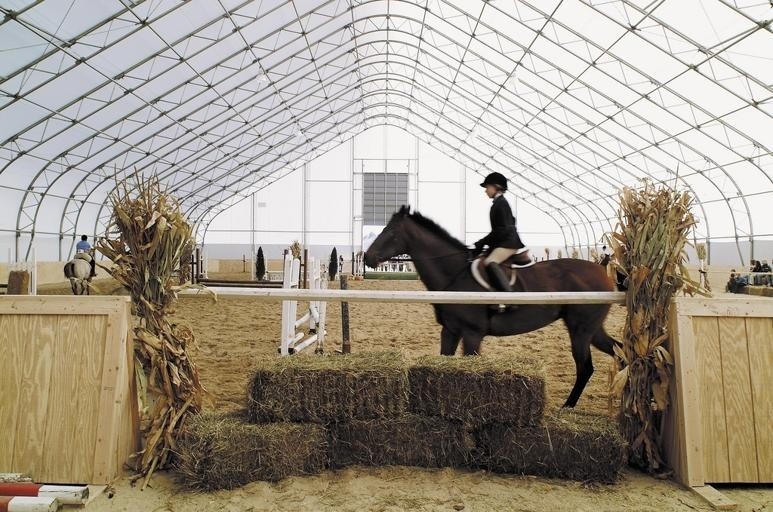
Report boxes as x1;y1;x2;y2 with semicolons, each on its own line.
82;235;87;240
480;172;507;188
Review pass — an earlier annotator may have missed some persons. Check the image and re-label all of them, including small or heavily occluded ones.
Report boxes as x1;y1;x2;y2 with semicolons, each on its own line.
473;172;525;312
599;245;607;264
727;259;773;293
74;235;96;276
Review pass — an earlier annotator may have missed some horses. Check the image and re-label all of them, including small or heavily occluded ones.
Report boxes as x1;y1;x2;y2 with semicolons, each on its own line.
62;247;97;295
360;205;629;410
601;252;612;267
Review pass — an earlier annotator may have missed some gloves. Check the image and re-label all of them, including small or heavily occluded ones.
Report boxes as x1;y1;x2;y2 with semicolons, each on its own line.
475;239;485;251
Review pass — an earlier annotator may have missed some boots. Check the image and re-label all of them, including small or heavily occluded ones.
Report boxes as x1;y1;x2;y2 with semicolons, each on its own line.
90;260;96;276
486;262;519;310
